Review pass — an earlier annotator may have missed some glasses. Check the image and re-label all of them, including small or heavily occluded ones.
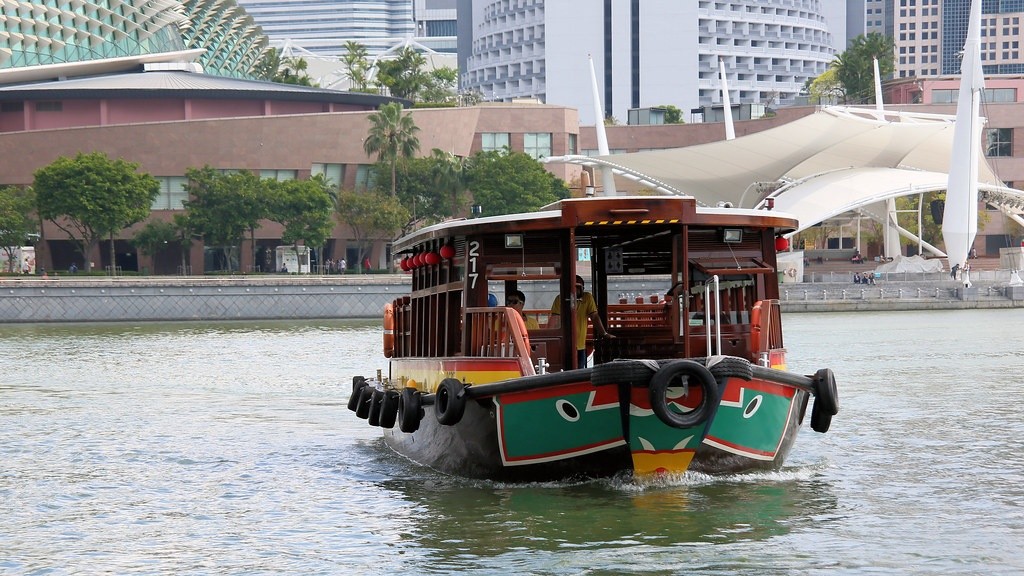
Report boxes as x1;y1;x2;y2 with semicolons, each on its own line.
506;298;524;306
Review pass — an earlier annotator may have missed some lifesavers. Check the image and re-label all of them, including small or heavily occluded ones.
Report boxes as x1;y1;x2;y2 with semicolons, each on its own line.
749;299;764;364
809;398;833;434
498;307;532;358
398;384;422;434
816;369;841;415
381;302;395;359
345;376;399;429
435;377;465;425
648;358;718;429
691;353;754;384
588;360;655;386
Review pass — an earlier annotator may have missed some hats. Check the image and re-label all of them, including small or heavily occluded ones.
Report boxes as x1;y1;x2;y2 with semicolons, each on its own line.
489;293;499;308
575;275;585;290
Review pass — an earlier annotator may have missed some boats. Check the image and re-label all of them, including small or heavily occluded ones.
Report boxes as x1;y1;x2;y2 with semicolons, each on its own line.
349;193;840;489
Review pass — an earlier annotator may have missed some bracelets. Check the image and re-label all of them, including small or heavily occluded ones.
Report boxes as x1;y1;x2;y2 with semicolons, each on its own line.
603;333;608;336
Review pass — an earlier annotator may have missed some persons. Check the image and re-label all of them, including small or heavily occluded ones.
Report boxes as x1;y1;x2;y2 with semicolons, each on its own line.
281;263;288;272
862;273;876;285
545;274;617;372
854;272;860;283
325;258;346;275
69;263;77;273
951;264;960;280
494;291;540;330
804;257;810;267
852;251;864;264
364;257;372;276
23;258;30;276
818;255;824;264
